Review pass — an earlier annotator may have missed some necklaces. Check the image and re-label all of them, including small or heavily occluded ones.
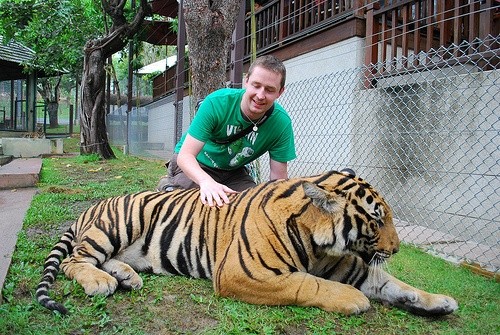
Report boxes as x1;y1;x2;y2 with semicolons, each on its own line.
240;91;266;132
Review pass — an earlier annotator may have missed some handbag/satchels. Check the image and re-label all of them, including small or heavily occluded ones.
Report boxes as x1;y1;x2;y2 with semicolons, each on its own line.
193;100;203;113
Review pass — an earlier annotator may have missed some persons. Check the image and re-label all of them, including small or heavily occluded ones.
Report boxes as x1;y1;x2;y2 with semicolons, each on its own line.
166;53;298;209
